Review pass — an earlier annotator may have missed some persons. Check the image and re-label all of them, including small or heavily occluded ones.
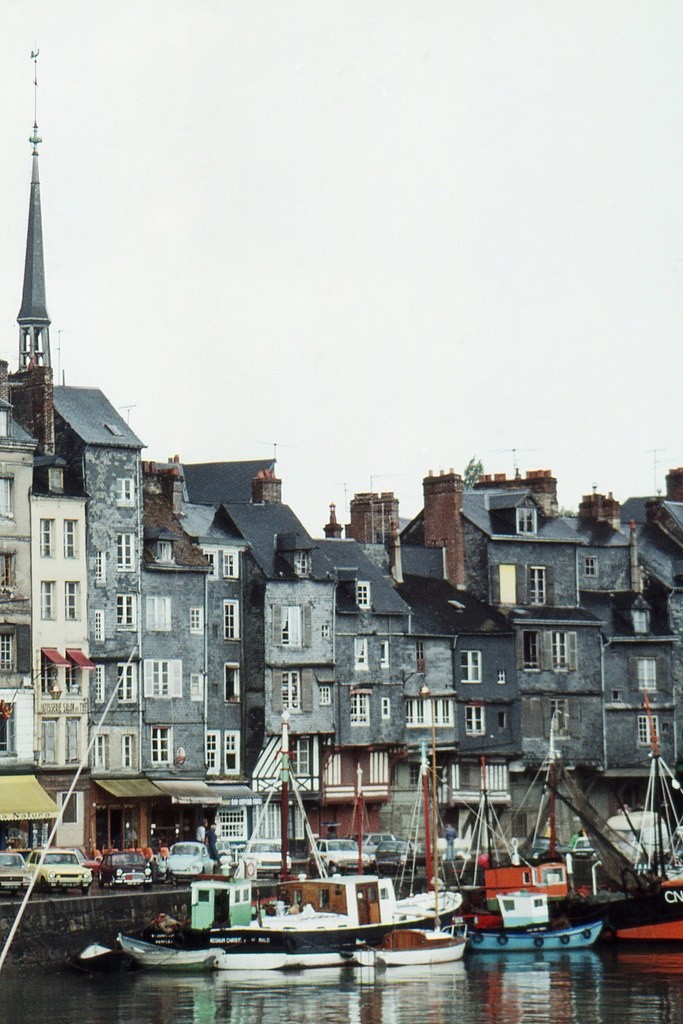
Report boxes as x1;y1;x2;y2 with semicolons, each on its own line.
445;824;458;861
327;827;337;839
196;819;220;862
142;913;173;943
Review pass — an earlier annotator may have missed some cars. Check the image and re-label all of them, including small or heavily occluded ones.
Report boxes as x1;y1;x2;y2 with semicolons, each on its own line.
165;841;214;883
373;841;416;874
26;848;92;894
573;837;600;858
309;839;369;878
0;853;32;897
240;839;291;877
522;836;565;866
351;833;396;864
98;853;152;890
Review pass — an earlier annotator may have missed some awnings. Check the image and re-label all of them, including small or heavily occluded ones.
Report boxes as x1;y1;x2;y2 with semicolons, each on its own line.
91;779;263;806
42;649;70;667
0;775;61;822
67;650;96;670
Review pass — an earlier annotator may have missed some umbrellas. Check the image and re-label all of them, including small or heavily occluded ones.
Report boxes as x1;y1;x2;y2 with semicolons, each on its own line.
320;820;341;827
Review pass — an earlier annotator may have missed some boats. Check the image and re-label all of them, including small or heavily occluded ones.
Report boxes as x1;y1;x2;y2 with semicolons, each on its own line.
340;929;466;966
445;889;602;952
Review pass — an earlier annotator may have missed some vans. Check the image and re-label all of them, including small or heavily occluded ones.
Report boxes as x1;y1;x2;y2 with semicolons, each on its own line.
601;813;670;866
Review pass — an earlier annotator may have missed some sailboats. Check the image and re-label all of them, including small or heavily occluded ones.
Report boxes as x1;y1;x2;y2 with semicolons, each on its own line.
117;710;468;972
449;692;683;949
